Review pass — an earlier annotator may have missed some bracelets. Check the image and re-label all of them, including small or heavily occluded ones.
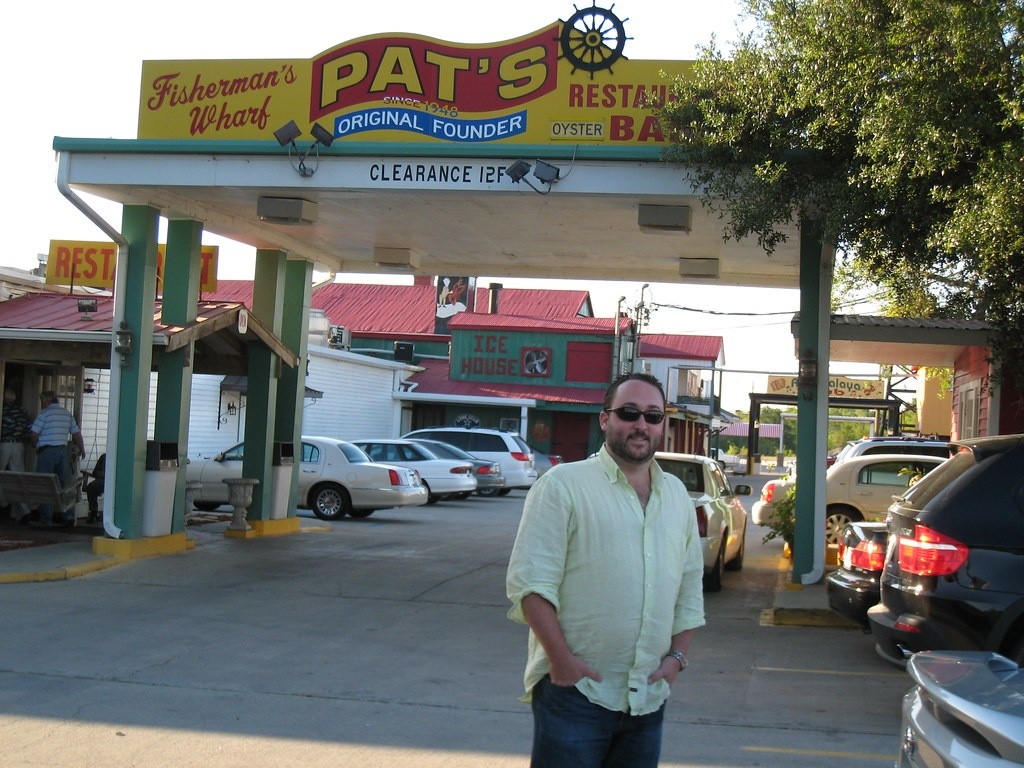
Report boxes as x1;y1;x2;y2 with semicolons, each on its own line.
666;650;688;672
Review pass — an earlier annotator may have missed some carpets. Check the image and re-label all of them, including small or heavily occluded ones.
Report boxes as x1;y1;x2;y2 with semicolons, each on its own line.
0;513;234;553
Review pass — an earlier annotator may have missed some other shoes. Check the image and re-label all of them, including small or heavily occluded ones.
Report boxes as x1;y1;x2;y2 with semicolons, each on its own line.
63;518;75;528
29;519;52;529
86;511;100;523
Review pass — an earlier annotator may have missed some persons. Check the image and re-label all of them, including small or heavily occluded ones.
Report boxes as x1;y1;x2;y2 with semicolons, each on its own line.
31;391;85;525
0;385;34;518
85;453;106;521
507;373;706;768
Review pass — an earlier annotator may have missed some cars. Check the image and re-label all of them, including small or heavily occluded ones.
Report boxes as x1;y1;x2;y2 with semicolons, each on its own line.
186;435;429;522
347;438;478;507
529;447;564;490
406;438;506;499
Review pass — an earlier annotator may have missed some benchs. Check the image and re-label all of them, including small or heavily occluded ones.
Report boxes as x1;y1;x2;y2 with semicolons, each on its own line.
0;469;99;529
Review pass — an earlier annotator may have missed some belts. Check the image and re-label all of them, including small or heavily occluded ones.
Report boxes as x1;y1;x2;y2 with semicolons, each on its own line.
1;438;24;442
39;445;66;452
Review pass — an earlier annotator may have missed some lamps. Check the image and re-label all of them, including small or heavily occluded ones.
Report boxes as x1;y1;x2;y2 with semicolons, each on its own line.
504;142;579;196
256;197;319;226
679;258;721;283
83;378;95;393
637;203;692;236
115;321;135;370
78;298;98;321
393;341;413;364
372;247;422;270
271;120;334;177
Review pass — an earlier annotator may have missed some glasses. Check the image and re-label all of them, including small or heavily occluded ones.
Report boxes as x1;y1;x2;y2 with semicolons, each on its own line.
605;406;665;424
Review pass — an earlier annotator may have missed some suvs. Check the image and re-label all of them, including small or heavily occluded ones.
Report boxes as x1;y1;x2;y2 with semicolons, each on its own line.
651;449;753;593
864;432;1024;675
394;425;539;498
751;427;959;633
